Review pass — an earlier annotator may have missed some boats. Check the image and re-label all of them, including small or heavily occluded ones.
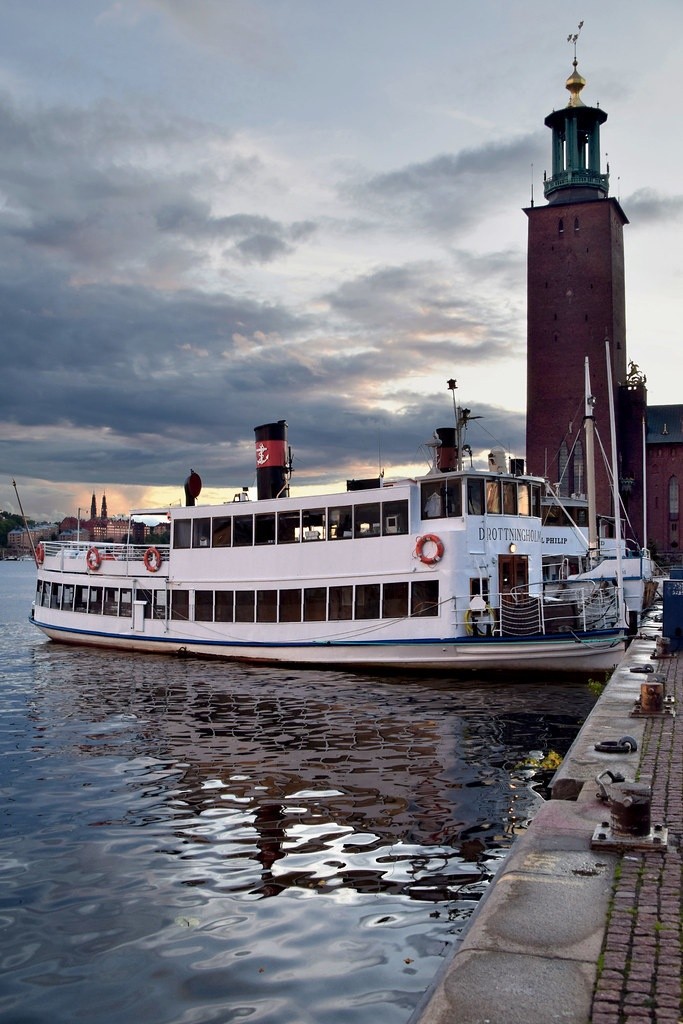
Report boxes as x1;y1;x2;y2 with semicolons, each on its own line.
27;378;653;672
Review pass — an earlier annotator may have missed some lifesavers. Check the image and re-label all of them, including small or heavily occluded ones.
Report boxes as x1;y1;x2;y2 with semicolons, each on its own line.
144;548;161;572
415;533;444;564
36;544;44;564
86;548;100;570
